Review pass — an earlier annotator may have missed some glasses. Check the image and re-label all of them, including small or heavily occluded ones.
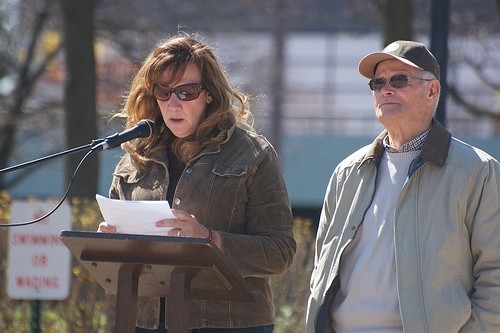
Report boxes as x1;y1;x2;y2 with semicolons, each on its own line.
369;75;431;92
152;83;207;101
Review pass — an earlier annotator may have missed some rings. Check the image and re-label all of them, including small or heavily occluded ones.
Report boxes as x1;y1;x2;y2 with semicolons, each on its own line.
97;31;298;333
177;229;181;237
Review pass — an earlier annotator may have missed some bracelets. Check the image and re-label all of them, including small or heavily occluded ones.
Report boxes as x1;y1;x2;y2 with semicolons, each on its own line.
205;226;217;242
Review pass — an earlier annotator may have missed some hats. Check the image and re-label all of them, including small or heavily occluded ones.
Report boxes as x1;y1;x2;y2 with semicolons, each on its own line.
358;41;440;81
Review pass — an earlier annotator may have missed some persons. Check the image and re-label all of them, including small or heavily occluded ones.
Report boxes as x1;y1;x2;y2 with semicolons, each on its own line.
304;40;500;333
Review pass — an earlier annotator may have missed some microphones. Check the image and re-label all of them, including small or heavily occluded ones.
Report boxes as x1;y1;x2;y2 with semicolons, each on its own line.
92;119;157;153
